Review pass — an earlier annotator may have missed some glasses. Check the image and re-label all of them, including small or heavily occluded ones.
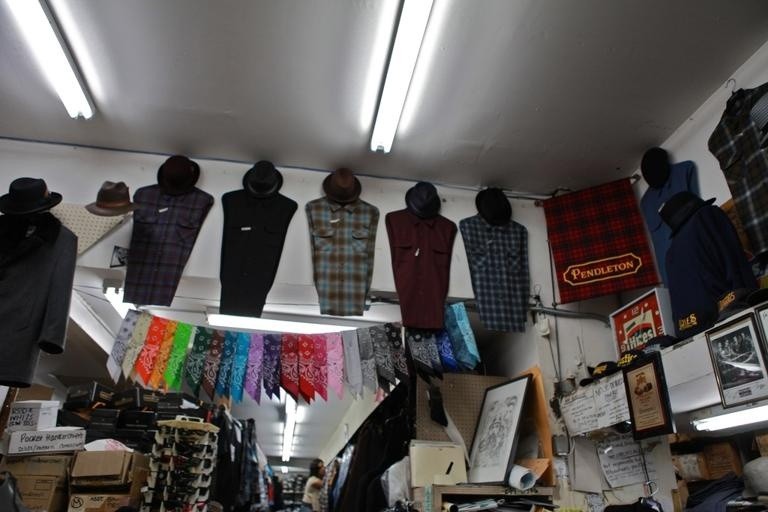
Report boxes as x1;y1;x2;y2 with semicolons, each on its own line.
204;458;211;466
209;432;218;442
152;438;169;450
169;486;197;495
206;446;216;454
148;468;166;479
202;473;212;482
166;501;194;511
177;444;205;453
199;486;210;495
172;472;200;482
198;500;210;510
181;429;209;436
150;453;169;462
144;484;163;497
142;500;159;509
157;425;172;436
174;457;201;466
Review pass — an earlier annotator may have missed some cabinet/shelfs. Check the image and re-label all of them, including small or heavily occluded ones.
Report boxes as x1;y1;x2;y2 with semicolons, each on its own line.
431;483;554;512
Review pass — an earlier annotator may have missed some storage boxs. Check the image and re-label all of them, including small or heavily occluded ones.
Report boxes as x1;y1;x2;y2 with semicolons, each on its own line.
0;454;72;512
71;451;148;496
0;427;85;454
68;494;140;512
8;401;60;432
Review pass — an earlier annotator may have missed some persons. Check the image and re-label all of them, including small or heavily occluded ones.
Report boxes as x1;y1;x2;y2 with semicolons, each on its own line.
300;459;326;512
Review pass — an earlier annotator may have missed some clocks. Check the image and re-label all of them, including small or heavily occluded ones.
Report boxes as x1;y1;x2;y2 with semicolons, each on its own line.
608;288;676;360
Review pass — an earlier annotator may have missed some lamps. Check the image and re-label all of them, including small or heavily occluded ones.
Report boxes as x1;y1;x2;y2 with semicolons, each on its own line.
365;0;433;152
5;0;100;119
204;306;357;335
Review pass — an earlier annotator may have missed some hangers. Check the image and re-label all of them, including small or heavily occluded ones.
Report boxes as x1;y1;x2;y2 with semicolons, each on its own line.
638;481;661;512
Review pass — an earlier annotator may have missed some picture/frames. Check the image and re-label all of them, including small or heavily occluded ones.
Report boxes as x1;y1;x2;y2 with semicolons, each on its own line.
754;303;768;373
704;312;768;409
466;373;533;484
623;352;673;441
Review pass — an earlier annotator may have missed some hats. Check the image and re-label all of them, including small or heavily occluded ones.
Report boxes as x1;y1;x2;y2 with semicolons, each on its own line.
658;191;717;238
243;161;283;198
713;289;760;327
616;350;646;371
322;168;362;203
641;148;671;189
0;178;62;215
84;181;139;216
476;188;512;225
406;182;441;219
643;335;676;355
580;361;618;386
676;312;713;342
157;156;200;195
